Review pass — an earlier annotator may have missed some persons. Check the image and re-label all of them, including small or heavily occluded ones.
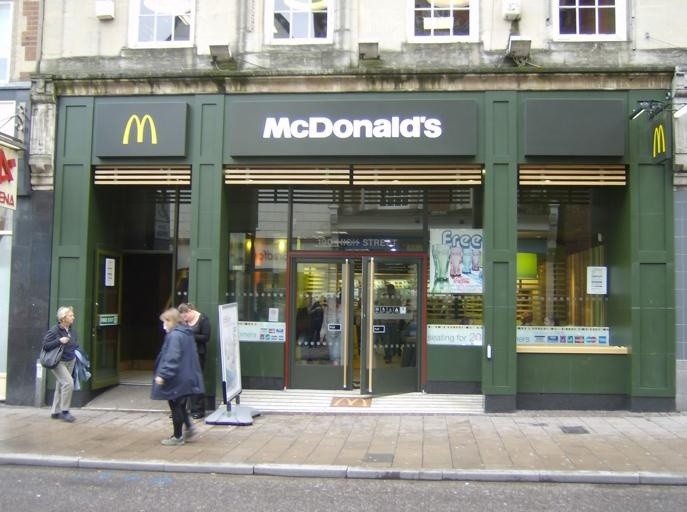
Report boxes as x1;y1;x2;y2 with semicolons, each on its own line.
520;312;532;326
296;284;416;366
149;307;206;446
40;307;81;422
460;318;474;325
176;301;210;419
544;315;560;326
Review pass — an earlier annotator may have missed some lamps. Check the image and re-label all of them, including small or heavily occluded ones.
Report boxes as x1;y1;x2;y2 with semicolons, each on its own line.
209;45;239;72
505;35;531;68
357;42;384;70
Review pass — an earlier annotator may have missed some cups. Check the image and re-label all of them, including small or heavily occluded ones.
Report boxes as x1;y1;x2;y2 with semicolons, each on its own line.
432;243;481;281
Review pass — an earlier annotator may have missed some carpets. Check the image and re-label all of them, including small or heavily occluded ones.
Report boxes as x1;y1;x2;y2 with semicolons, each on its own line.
329;397;372;408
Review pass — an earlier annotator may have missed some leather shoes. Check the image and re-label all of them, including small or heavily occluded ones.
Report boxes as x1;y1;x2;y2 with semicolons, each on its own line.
62;413;77;422
193;414;203;419
51;413;64;420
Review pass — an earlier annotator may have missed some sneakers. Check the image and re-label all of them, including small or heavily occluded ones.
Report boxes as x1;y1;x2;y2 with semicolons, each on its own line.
161;436;184;446
184;430;201;443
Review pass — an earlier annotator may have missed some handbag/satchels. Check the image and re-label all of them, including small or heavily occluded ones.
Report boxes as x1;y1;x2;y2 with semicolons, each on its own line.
39;344;64;368
